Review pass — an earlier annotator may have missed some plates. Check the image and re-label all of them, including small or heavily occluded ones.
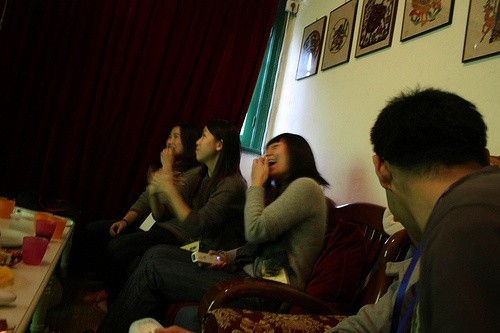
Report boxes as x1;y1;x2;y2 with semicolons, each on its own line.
0;248;23;268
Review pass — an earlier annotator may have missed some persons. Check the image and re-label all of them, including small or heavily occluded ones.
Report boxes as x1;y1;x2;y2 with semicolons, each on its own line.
75;89;500;333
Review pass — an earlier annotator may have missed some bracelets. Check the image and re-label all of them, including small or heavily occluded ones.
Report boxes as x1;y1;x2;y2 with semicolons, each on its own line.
122;218;129;228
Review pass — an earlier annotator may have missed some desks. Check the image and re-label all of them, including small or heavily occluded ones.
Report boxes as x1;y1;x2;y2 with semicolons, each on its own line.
0;207;74;333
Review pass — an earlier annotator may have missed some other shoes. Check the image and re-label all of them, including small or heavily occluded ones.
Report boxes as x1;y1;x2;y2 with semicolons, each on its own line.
83;288;108;306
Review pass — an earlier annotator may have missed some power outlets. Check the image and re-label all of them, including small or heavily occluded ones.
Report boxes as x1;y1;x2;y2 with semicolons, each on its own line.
286;1;298;14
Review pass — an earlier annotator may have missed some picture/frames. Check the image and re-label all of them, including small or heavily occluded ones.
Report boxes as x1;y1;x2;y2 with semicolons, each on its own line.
461;0;500;64
320;0;359;71
400;0;455;42
354;0;398;58
295;15;328;81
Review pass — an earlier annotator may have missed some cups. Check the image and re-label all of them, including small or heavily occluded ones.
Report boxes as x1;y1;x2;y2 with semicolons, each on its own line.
53;216;67;240
0;198;16;219
33;212;55;241
21;236;50;265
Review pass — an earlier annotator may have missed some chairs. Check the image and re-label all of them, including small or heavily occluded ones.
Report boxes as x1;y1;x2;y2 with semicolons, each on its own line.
194;198;412;333
